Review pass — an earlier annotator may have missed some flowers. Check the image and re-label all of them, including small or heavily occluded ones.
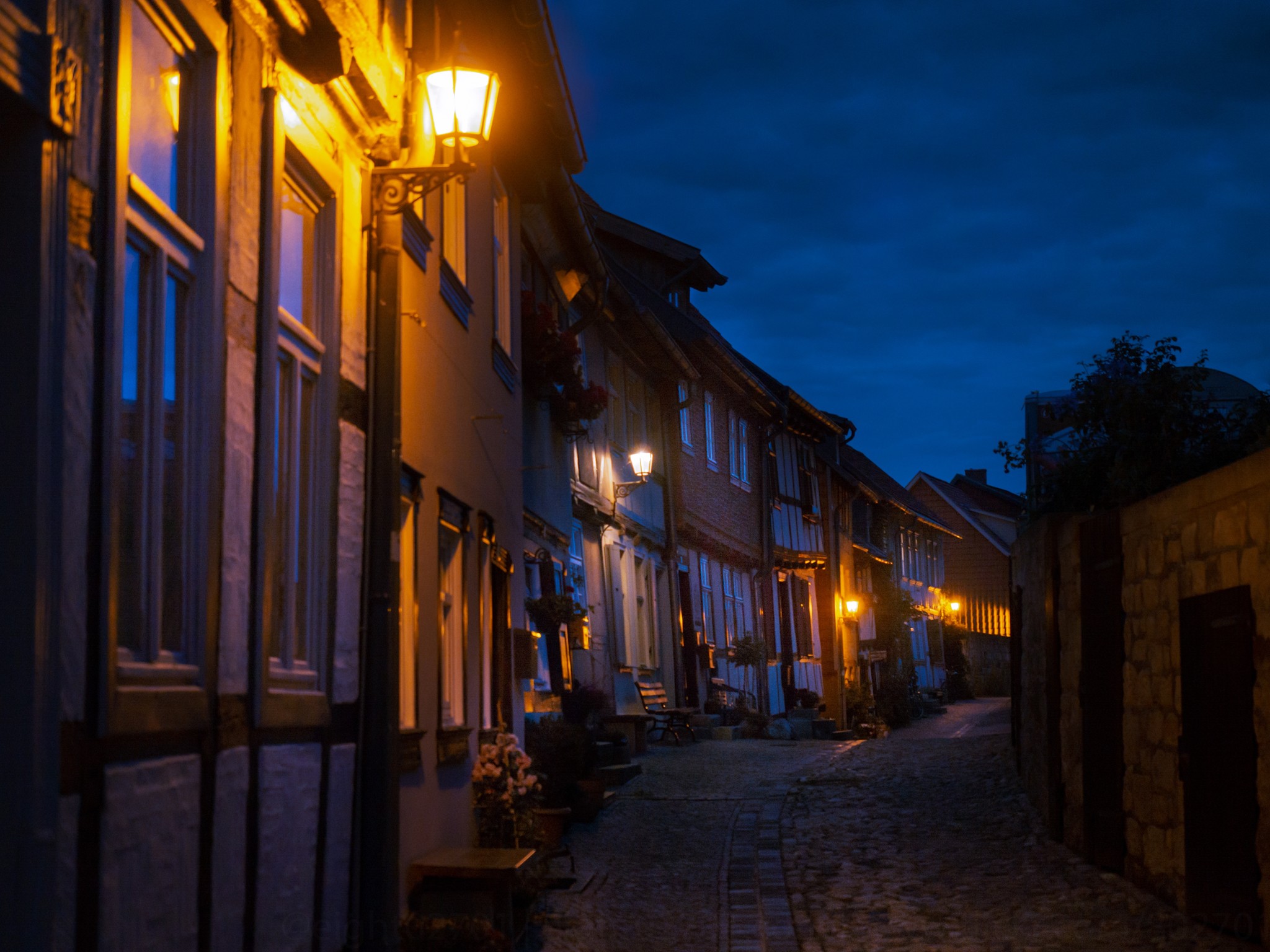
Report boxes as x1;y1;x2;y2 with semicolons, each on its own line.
532;294;608;429
470;731;542;819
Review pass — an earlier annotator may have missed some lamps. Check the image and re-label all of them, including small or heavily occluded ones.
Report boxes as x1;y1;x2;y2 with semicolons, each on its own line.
401;30;504;211
612;443;653;518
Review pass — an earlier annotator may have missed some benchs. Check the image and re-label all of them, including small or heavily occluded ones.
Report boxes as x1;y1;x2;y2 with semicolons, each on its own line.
634;681;702;744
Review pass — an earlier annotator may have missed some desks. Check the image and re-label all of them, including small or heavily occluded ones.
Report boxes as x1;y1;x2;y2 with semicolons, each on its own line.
410;845;535;952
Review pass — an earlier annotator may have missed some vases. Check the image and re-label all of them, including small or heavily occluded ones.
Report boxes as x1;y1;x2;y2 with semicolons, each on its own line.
534;807;572;854
